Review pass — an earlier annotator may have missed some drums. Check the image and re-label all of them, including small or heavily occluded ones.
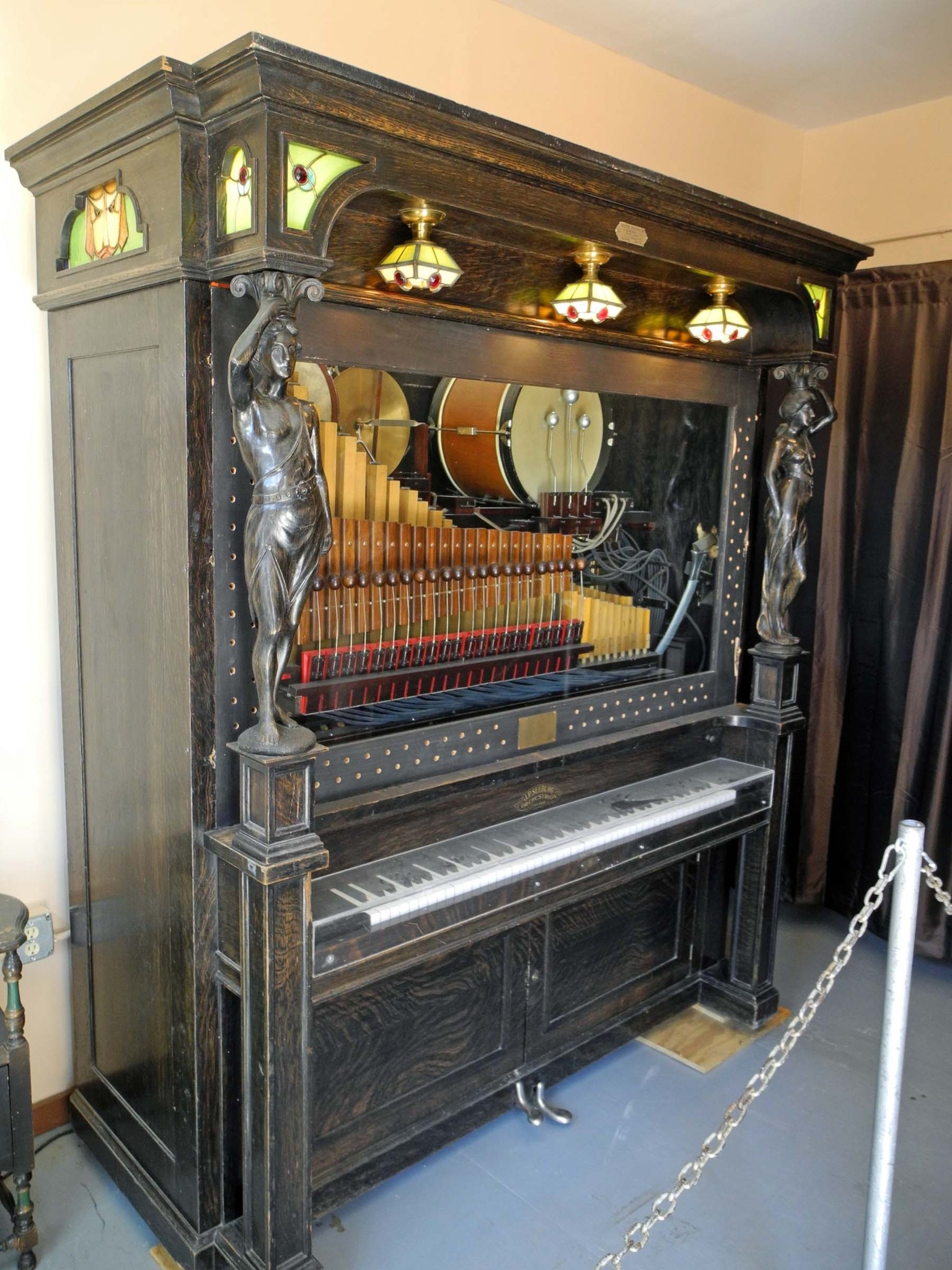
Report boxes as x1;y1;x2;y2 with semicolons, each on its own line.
429;376;614;509
292;362;340;424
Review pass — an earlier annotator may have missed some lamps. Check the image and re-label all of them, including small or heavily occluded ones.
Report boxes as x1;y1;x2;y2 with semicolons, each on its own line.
551;236;629;329
683;281;754;349
369;196;465;301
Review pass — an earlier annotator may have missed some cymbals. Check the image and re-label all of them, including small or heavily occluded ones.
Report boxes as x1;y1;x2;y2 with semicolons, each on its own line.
333;366;410;476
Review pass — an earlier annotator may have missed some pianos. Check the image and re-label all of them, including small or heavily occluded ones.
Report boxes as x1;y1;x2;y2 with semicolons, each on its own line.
6;31;873;1270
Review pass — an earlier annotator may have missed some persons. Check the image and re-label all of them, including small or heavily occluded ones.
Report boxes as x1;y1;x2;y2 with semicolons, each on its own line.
756;363;838;645
228;270;334;746
